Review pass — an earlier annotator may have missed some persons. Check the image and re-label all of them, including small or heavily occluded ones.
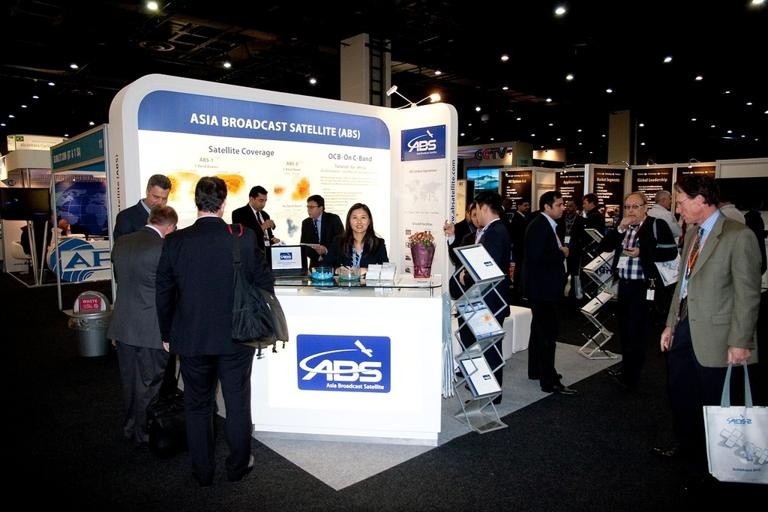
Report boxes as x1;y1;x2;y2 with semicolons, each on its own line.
601;174;767;488
59;218;94;240
443;191;605;406
33;207;54;285
111;174;174;241
231;186;281;271
299;195;344;272
328;201;387;277
154;176;278;486
104;204;179;449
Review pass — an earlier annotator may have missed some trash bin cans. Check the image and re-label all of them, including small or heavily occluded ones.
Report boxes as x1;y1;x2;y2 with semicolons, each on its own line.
69;291;111;357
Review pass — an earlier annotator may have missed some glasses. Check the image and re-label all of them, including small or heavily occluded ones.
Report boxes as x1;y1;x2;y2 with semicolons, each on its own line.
623;204;645;209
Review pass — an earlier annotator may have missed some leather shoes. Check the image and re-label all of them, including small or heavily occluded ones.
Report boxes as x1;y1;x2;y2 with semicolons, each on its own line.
247;454;254;472
653;445;679;458
542;384;578;397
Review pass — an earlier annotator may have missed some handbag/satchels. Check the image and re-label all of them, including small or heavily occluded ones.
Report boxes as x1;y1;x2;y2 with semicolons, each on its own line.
146;394;219;460
231;285;288;348
702;405;768;485
652;218;682;287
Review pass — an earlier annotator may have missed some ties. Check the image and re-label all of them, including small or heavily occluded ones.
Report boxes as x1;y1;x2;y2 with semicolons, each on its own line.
256;212;262;225
679;226;703;322
314;219;319;240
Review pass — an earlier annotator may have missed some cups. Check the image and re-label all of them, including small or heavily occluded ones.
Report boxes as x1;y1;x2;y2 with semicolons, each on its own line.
339;266;361;282
311;266;334;286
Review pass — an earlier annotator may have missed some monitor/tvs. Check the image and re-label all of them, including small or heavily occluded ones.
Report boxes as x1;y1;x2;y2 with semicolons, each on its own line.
266;245;308;277
0;188;51;220
466;165;504;195
